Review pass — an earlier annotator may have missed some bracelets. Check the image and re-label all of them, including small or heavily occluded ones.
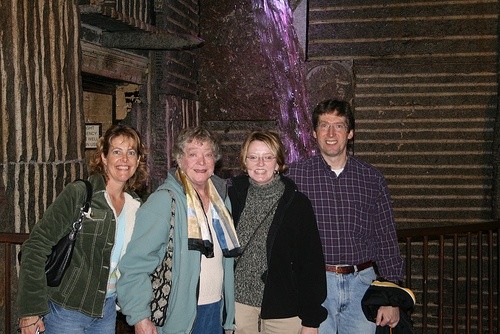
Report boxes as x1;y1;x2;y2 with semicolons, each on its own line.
19;318;39;327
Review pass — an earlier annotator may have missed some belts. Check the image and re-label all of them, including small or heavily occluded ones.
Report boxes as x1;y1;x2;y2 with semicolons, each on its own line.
325;261;372;274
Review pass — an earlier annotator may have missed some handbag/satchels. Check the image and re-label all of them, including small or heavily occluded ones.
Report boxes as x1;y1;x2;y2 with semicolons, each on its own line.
17;179;93;288
115;189;176;327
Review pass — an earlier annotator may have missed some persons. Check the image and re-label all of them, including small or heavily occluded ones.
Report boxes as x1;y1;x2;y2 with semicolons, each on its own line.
16;126;149;334
116;126;241;334
287;99;404;334
229;130;328;334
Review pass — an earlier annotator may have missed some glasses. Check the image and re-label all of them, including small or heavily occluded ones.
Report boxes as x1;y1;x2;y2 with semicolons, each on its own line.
316;122;348;130
246;155;278;162
